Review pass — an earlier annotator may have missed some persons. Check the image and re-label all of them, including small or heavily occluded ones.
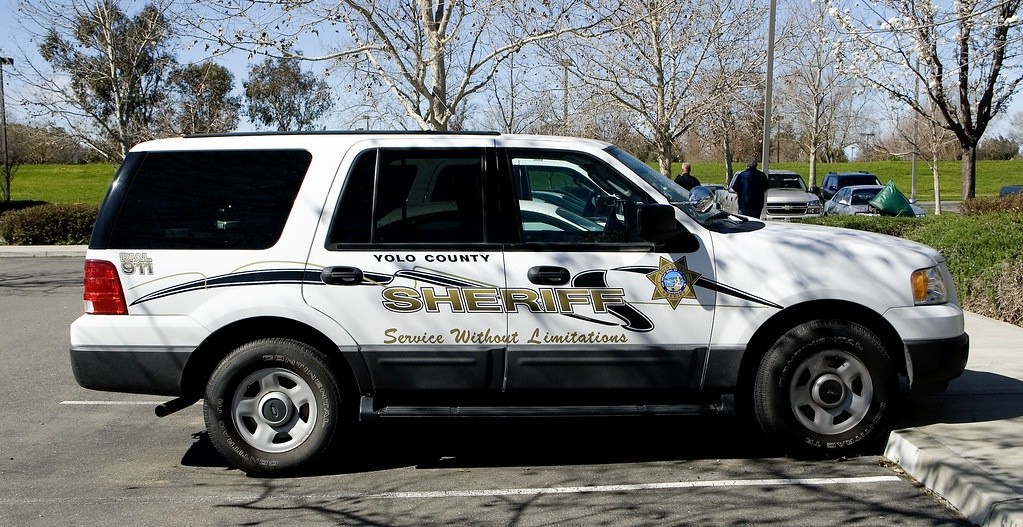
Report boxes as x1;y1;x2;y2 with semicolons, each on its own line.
727;155;769;218
674;162;701;194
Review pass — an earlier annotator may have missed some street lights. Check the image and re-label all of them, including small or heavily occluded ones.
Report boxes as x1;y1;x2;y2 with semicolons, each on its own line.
776;116;784;163
850;147;855;162
0;57;16;204
560;57;574;136
363;115;371;131
858;132;874;162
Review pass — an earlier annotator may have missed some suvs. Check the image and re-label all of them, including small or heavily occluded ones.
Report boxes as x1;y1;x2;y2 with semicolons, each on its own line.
819;171;884;206
68;127;973;480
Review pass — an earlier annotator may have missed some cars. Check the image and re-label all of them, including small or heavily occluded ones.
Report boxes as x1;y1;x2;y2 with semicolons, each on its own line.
823;184;925;219
700;183;726;210
715;169;824;222
